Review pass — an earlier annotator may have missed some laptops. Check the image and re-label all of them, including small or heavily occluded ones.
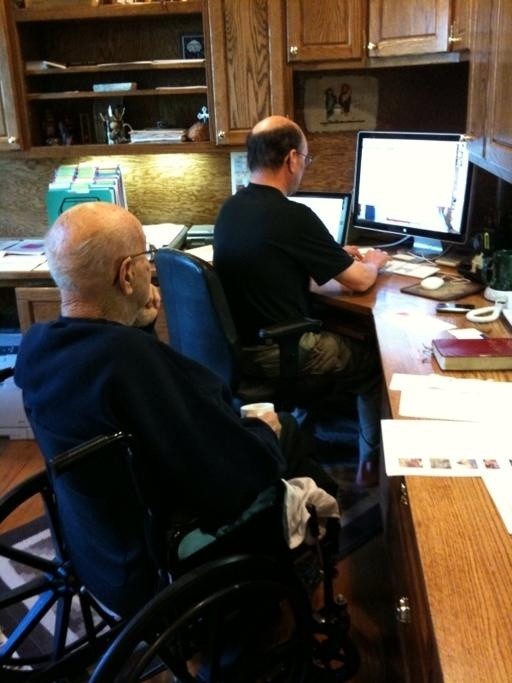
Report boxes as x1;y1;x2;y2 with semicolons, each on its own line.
286;192;351;246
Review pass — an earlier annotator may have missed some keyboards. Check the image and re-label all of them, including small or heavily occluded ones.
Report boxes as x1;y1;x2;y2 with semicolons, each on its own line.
361;255;441;279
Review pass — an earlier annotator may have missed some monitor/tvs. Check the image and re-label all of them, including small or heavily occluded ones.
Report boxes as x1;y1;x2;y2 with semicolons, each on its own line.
352;131;478;255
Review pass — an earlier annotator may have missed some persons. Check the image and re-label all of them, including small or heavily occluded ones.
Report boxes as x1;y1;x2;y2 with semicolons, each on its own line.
12;197;341;681
211;112;396;494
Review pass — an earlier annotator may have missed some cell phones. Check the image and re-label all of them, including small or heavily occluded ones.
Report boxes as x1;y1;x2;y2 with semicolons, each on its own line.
436;303;474;312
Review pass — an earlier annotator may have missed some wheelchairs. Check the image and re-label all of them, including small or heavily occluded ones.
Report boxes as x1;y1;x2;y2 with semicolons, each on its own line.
0;369;383;682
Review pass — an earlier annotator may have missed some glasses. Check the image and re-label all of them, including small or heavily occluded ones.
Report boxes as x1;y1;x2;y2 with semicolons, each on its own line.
112;243;158;287
286;150;313;168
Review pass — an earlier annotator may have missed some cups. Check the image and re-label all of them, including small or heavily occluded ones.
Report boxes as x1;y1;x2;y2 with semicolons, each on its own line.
104;114;133;145
485;249;512;292
240;401;275;423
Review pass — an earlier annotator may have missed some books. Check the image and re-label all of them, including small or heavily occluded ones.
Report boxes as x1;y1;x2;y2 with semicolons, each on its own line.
152;57;207;66
97;61;153;67
128;127;187;144
432;335;511;375
0;162;216;272
155;85;208;92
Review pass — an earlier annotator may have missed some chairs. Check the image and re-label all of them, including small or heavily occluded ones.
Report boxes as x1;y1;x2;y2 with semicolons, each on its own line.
154;248;369;462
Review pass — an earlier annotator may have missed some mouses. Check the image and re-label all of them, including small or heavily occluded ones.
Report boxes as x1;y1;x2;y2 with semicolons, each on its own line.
421;277;444;290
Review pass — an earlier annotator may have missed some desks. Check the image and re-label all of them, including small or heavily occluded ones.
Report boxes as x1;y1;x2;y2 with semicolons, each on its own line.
181;244;512;683
0;223;186;334
